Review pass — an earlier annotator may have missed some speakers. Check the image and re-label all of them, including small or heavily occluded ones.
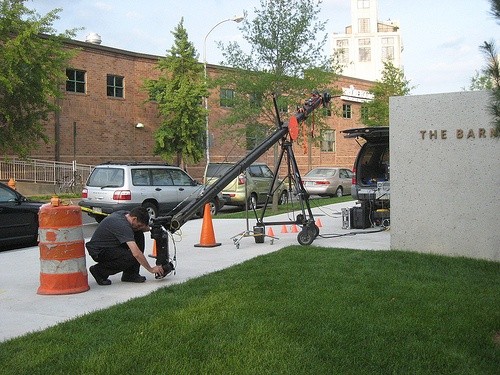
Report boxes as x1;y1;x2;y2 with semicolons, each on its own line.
353;206;371;228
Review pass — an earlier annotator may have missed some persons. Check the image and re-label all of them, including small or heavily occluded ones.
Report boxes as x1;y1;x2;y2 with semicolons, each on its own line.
86;207;164;285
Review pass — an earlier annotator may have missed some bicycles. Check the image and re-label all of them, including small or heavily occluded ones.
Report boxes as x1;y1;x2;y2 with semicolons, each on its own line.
54;174;84;196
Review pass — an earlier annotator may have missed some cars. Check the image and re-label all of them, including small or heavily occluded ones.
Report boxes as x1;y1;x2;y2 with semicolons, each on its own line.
301;167;352;200
0;182;45;252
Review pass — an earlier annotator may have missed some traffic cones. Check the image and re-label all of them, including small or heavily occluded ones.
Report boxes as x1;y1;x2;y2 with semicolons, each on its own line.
316;218;322;227
148;240;157;258
268;227;275;236
291;225;299;233
194;204;222;248
281;225;288;233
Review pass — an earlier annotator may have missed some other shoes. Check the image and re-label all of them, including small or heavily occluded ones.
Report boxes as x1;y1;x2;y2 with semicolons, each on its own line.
121;273;147;283
89;265;111;286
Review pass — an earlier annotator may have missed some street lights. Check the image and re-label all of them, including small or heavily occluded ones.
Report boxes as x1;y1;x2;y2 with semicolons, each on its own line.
203;15;244;164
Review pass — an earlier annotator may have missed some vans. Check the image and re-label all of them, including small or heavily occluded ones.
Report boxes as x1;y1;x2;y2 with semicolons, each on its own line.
339;126;390;210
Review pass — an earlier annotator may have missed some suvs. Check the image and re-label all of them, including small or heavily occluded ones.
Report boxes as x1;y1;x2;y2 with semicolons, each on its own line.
202;161;289;211
78;160;225;225
